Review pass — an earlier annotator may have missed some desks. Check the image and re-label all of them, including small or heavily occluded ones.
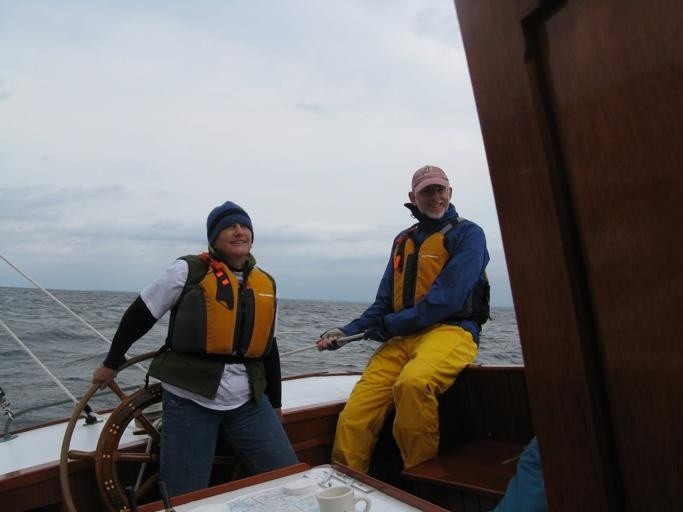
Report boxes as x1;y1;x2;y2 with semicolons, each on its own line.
123;459;448;512
400;435;523;512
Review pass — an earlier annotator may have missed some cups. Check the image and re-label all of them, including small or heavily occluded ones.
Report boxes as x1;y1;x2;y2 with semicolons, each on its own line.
317;485;370;512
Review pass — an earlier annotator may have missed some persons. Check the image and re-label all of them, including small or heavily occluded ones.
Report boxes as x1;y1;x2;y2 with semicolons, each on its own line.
315;164;489;477
89;200;299;499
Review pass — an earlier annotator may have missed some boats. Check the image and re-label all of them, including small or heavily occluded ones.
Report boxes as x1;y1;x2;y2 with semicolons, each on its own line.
0;0;683;511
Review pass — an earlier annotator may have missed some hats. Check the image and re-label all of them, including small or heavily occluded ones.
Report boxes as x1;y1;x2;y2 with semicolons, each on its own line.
412;165;449;193
207;201;253;248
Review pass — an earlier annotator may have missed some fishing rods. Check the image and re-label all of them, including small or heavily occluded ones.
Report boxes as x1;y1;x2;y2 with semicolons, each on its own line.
280;332;366;358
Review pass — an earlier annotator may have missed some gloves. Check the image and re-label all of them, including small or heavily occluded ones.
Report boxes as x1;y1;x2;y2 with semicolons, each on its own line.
363;322;390;343
